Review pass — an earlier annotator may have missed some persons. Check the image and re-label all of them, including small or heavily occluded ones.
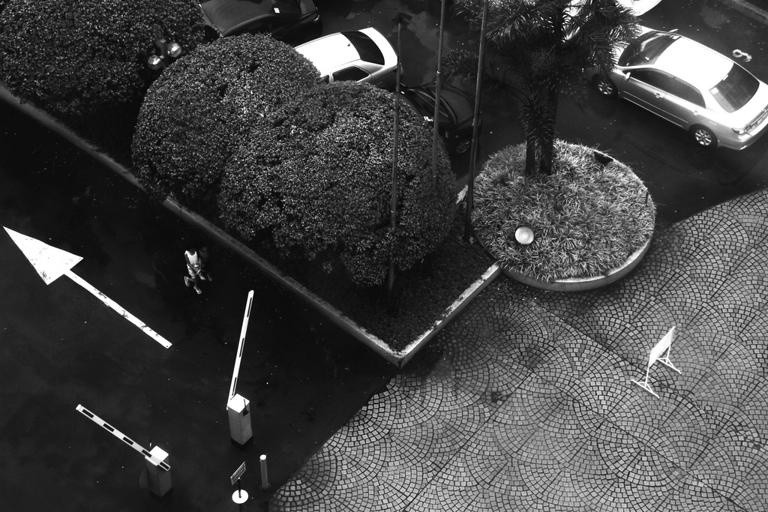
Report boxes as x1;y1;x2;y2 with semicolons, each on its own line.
183;240;206;296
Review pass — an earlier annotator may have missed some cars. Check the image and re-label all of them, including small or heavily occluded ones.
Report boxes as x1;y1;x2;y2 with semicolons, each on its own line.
390;80;481;158
596;0;663;20
581;21;768;152
479;1;594;47
293;27;404;91
139;1;321;81
